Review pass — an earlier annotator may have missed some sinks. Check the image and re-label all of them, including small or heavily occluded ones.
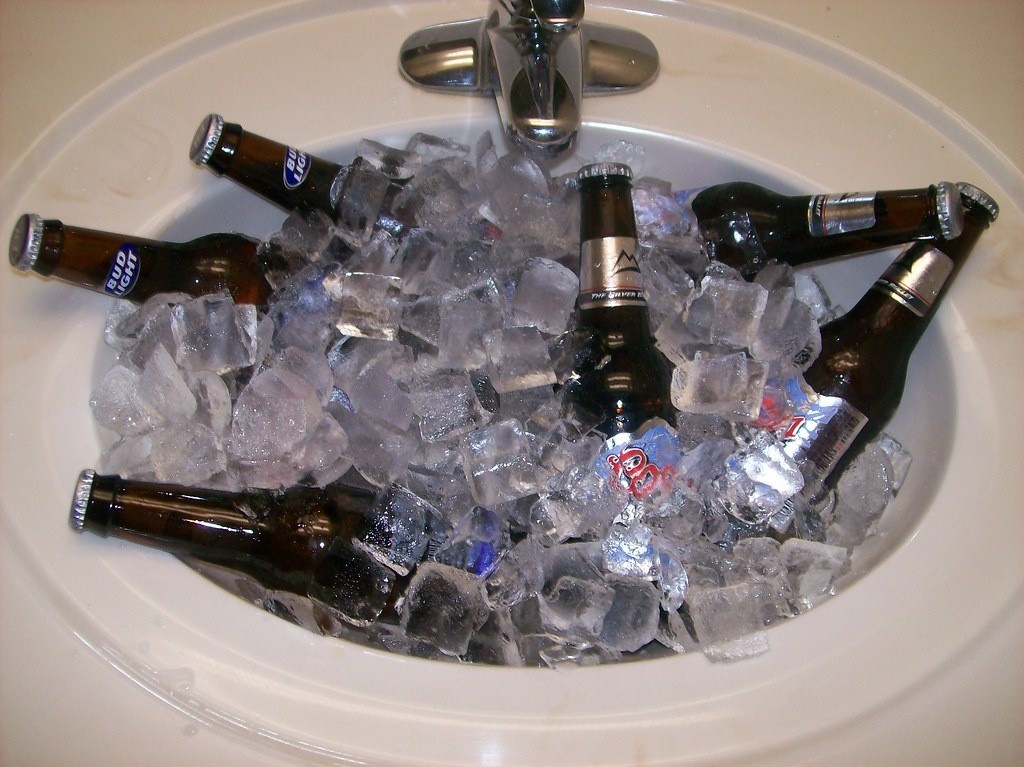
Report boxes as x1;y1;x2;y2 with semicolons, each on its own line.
0;5;1023;767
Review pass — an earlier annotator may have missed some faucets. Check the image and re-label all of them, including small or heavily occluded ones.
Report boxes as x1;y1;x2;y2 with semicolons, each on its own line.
397;0;661;164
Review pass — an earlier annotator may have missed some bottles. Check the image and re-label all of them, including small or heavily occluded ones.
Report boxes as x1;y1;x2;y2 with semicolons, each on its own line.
547;161;683;617
669;182;963;283
711;182;999;548
188;113;425;254
68;469;505;643
9;213;314;343
